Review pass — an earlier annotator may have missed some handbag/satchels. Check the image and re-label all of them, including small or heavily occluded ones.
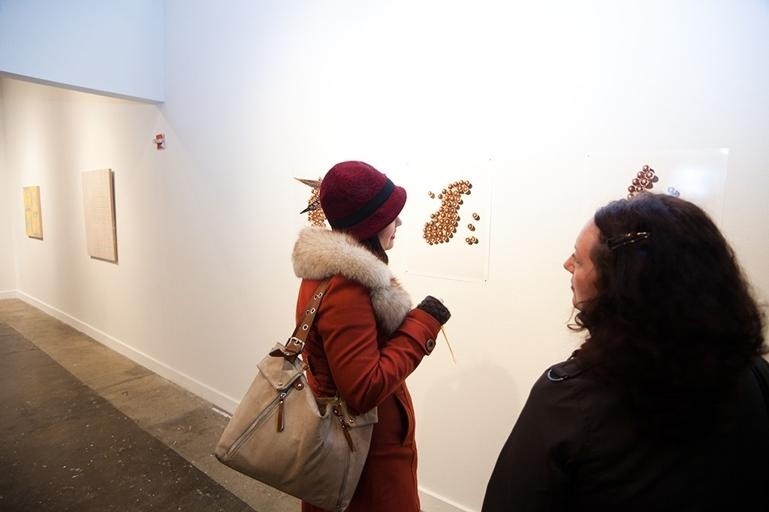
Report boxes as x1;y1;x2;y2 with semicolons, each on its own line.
215;276;377;512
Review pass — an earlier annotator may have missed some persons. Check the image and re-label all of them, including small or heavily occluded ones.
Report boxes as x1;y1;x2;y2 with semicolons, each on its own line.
293;161;450;511
481;191;768;512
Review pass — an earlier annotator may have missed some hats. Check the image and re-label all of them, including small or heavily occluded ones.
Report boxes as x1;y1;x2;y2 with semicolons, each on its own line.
319;162;407;242
